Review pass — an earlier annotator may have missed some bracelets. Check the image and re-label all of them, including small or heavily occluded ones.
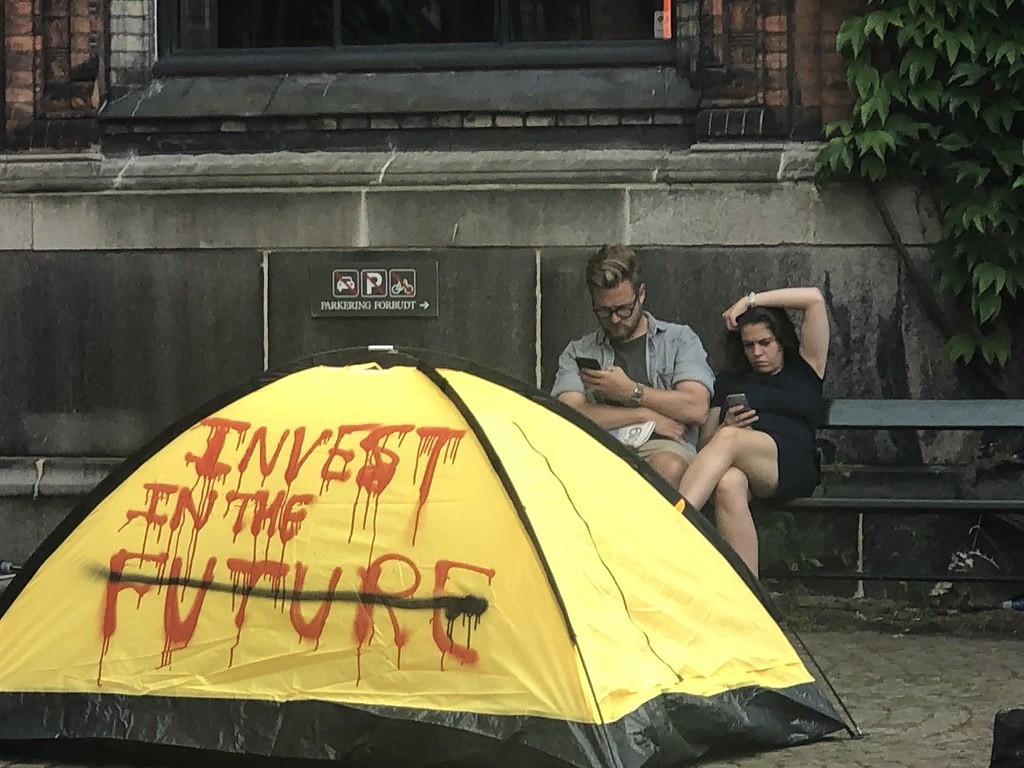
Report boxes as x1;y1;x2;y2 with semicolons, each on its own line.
631;383;645;407
749;292;755;308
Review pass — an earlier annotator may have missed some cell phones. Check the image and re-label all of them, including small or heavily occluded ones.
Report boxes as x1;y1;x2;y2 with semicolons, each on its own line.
575;357;602;385
726;392;754;427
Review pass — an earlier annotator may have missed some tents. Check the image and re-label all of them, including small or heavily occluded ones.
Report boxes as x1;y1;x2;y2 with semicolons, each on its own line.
0;345;866;768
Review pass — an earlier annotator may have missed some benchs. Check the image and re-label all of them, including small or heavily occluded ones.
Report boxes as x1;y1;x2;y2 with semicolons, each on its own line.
784;399;1024;509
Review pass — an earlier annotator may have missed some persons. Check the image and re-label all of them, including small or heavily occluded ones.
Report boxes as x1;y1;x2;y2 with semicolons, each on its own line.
549;245;715;493
680;287;829;577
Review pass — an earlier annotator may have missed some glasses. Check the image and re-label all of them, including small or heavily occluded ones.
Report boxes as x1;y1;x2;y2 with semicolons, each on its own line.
592;294;638;319
